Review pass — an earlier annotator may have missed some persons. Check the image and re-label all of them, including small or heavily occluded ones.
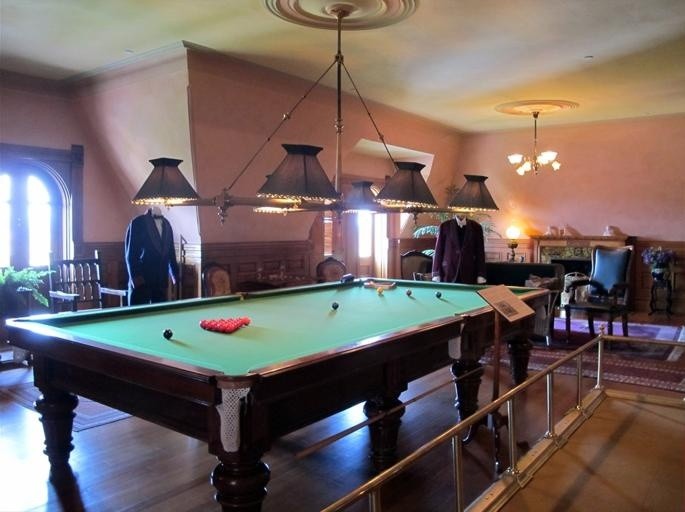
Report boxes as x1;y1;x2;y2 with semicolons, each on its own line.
431;212;487;285
125;207;179;306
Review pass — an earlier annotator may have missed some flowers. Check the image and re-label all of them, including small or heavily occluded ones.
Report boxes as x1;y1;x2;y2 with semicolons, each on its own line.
644;246;681;266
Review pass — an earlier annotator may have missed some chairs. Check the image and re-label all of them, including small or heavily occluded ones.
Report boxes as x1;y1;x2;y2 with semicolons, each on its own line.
49;250;128;313
203;262;232;297
314;257;347;282
562;244;634;344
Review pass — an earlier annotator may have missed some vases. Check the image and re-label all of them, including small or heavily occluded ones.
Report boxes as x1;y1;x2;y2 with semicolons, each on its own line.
652;262;670;281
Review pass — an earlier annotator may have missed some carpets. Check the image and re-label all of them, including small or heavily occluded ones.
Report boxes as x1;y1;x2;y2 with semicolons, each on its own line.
4;383;134;432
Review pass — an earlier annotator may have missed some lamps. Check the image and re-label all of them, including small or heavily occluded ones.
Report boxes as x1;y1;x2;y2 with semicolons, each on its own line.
131;1;496;212
495;100;581;179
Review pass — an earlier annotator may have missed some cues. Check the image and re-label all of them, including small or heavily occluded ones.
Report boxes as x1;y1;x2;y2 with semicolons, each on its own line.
295;365;488;460
179;235;184;300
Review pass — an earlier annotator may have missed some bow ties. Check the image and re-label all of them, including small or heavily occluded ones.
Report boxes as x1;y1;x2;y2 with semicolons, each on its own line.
154;215;163;219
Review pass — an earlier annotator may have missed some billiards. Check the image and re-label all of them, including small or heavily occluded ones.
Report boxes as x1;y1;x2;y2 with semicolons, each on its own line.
378;288;382;293
406;290;412;296
332;302;339;309
198;317;250;333
436;292;442;298
164;330;172;339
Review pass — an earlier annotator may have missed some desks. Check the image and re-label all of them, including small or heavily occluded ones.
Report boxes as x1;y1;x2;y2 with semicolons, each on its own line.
649;279;672;320
5;277;551;510
